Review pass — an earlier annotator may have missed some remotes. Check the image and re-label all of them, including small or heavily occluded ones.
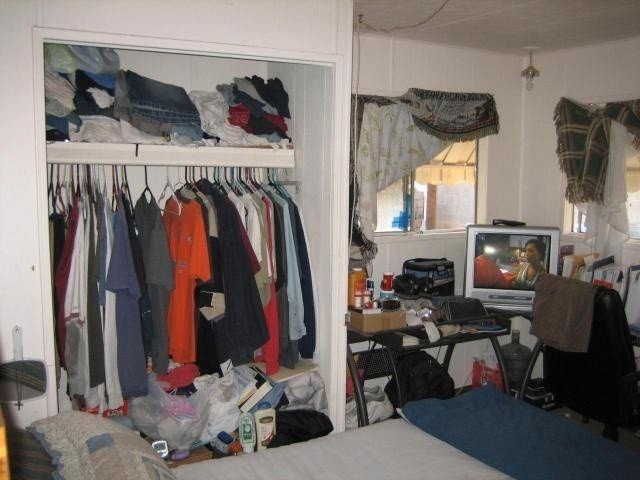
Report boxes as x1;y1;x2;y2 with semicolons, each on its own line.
493;220;526;227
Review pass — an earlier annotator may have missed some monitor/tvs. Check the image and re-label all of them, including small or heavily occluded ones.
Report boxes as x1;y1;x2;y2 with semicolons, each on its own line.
464;227;560;311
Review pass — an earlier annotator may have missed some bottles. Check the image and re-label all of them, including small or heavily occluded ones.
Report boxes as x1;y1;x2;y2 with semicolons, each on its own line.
349;268;366;305
367;280;374;301
354;290;362;307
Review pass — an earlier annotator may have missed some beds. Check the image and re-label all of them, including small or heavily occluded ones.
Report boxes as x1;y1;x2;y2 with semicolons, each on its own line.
0;383;640;480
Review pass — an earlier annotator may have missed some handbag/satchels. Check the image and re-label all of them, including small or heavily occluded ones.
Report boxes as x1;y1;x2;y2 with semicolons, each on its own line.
440;295;488;321
511;377;562;410
383;351;454;418
402;258;455;295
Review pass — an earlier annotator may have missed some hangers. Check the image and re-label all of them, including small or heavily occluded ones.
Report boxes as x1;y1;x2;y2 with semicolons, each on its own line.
48;162;296;223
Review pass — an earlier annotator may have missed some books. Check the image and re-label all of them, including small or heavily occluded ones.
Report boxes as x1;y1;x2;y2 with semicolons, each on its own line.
232;358;319;412
560;242;640;327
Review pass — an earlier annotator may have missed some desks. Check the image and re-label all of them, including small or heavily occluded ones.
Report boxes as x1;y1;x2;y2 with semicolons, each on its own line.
347;307;511;416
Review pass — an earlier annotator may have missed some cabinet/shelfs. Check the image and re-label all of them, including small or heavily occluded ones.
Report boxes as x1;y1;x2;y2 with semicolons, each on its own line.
1;0;353;464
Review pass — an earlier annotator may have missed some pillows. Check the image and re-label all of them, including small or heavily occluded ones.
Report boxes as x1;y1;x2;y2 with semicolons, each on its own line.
25;411;181;480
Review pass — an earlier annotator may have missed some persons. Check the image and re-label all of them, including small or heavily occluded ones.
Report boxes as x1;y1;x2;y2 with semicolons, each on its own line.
516;239;547;290
475;234;519;290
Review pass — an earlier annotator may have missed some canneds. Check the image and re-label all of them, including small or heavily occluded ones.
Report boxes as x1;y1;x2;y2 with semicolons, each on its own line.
354;291;362;309
380;289;396;301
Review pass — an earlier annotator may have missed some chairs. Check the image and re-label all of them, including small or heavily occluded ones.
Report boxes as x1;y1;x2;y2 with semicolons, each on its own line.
529;272;640;443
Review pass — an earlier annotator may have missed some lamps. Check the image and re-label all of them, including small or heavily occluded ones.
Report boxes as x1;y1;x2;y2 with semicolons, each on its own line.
520;47;539;91
